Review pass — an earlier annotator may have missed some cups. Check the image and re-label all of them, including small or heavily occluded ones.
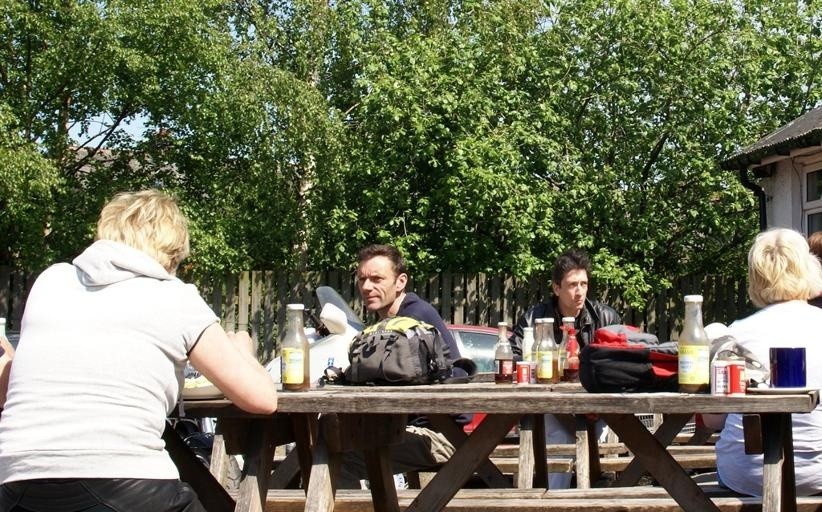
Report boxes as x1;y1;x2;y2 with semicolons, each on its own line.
769;346;806;387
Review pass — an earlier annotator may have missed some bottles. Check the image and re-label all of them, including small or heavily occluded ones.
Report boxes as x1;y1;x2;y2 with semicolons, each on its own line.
534;317;558;383
515;360;532;386
559;316;581;383
280;303;311;392
675;295;711;394
493;321;513;384
726;355;748;398
521;327;534;361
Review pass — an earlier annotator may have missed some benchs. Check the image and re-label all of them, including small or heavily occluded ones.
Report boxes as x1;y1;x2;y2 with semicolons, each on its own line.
225;431;822;510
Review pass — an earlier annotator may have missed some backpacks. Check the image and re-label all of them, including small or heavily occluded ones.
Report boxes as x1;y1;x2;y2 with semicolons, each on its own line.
578;325;681;393
346;316;454;386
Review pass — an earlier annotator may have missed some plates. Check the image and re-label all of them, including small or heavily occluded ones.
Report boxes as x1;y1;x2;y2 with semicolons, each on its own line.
747;387;819;395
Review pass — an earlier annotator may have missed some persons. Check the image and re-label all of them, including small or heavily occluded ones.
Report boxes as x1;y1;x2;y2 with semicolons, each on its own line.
501;241;627;492
0;183;281;512
314;244;478;494
707;224;822;504
